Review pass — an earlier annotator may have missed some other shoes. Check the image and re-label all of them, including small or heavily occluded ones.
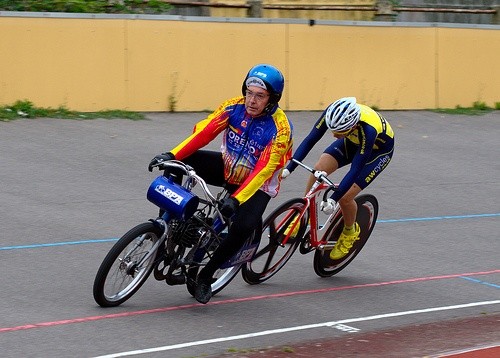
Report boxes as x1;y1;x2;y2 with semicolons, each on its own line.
195;268;211;303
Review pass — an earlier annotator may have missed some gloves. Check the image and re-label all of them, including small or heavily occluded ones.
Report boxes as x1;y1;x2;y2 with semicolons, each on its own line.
148;152;175;172
218;198;239;218
320;197;336;215
277;168;291;180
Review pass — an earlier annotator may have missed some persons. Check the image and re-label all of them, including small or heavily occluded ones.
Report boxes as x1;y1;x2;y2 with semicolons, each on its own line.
146;64;293;304
280;96;395;260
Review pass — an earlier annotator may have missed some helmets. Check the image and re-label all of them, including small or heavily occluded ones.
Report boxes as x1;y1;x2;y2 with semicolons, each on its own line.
242;63;284;115
326;97;361;131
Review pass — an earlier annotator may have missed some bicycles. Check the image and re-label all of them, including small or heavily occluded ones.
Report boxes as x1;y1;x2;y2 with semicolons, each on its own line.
92;158;263;307
241;157;379;285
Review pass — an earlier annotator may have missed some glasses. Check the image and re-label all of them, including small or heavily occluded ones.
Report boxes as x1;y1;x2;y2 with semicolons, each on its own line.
245;90;270;100
331;126;356;136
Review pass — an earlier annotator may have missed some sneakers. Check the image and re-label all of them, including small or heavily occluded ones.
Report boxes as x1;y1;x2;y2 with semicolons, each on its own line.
329;221;361;259
283;212;311;238
